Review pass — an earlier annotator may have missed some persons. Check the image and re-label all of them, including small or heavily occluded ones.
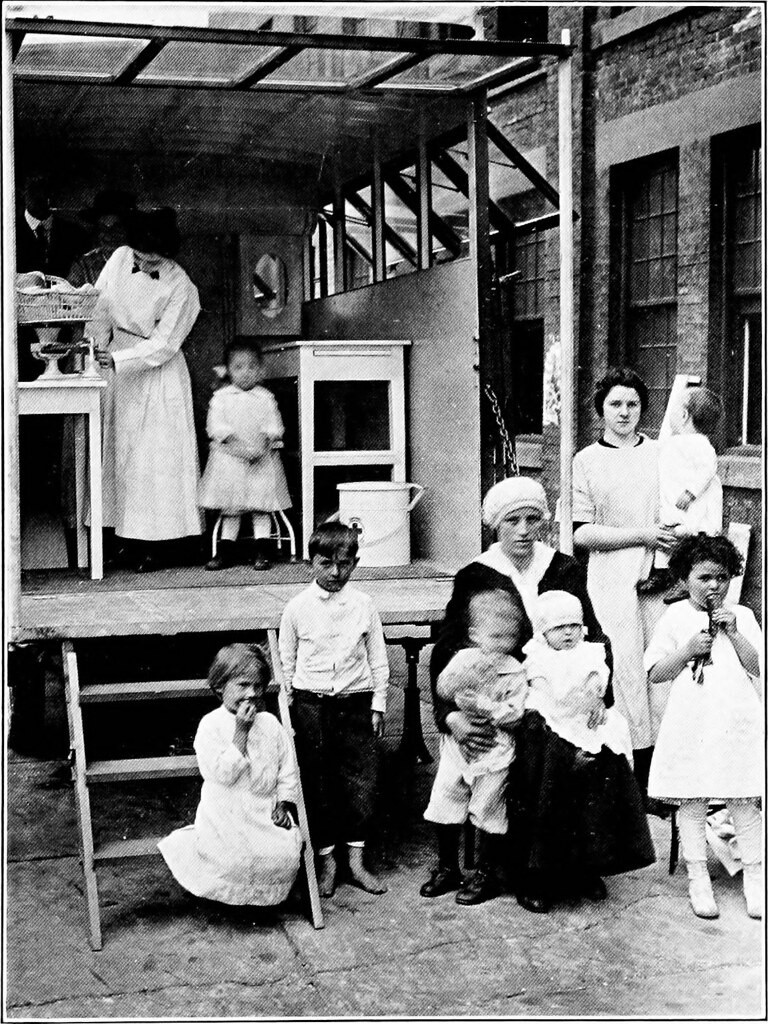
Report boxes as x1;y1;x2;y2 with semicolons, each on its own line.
419;367;762;918
156;643;302;906
66;213;124;371
277;521;390;898
81;207;207;573
196;335;292;572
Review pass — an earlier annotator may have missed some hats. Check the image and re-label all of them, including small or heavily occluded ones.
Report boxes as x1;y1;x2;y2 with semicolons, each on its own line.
530;589;582;635
481;476;549;530
118;205;185;258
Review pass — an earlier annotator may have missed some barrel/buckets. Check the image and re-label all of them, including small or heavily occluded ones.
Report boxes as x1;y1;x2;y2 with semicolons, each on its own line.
327;480;424;567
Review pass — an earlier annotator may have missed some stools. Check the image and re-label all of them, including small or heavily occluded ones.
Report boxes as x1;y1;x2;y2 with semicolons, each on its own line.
210;440;297;566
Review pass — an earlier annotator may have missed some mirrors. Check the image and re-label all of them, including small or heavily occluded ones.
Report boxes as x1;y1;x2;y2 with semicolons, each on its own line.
253;252;287;318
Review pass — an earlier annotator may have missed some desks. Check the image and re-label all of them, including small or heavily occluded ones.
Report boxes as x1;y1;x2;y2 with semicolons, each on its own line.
258;338;412;560
17;379;107;581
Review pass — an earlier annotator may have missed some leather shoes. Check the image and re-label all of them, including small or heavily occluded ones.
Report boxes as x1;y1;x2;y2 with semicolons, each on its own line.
580;878;608;899
420;867;461;896
514;881;551;913
455;874;498;904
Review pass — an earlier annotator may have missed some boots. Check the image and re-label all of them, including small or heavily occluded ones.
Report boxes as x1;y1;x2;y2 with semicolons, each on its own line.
207;539;236;569
255;537;272;570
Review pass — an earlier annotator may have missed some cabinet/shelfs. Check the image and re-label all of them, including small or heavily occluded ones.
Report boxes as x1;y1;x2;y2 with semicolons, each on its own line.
237;234;303;336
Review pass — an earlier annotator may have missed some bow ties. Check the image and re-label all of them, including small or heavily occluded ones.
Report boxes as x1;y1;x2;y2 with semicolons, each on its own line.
131;263;160;279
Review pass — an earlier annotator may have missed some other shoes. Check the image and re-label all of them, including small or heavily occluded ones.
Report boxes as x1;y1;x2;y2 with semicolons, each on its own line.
663;577;689;604
742;876;764;917
638;569;675;595
689;883;720;917
